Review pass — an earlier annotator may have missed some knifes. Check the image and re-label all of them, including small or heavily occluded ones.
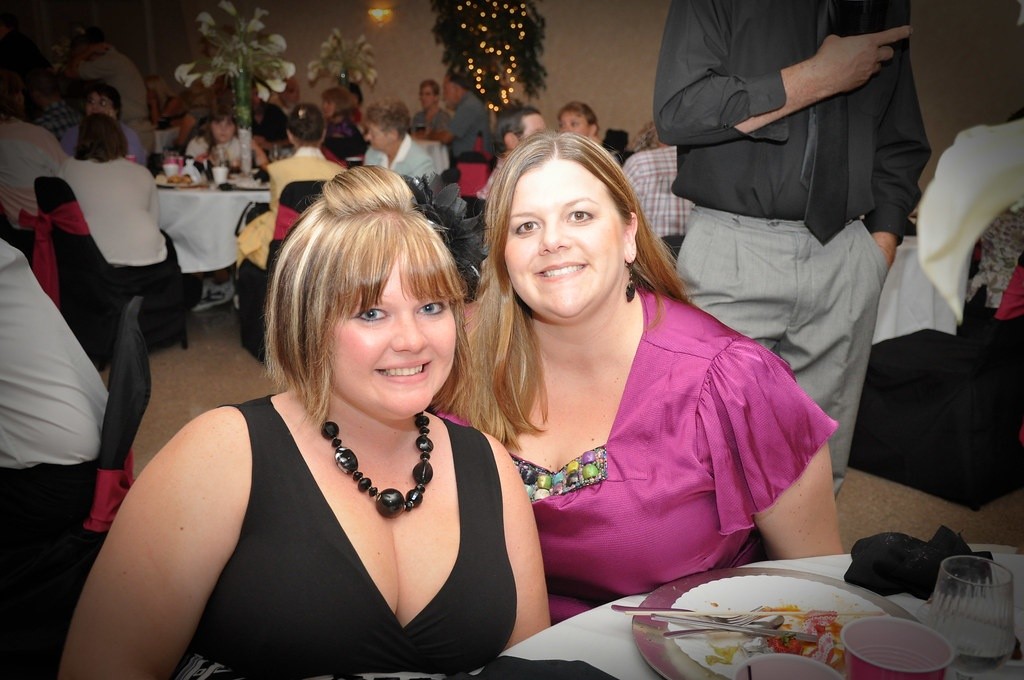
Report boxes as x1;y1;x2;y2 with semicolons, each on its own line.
649;613;819;644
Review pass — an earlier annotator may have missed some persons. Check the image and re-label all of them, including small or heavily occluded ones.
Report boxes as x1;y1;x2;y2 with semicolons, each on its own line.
150;71;491;312
654;0;933;499
0;12;198;267
59;164;552;680
427;131;844;623
477;101;697;261
0;237;107;544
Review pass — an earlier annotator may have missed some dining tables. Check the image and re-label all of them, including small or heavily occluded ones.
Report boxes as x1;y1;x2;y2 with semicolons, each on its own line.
152;178;271;311
465;540;1024;680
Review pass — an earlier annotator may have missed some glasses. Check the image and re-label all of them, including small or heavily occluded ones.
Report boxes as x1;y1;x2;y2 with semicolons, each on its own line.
87;99;111;107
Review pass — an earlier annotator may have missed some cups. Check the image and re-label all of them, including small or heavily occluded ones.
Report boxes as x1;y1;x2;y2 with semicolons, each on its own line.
167;156;183;175
211;166;229;186
840;616;955;680
162;163;179;179
268;142;281;163
210;146;227;164
125;155;137;163
923;556;1017;680
734;653;845;680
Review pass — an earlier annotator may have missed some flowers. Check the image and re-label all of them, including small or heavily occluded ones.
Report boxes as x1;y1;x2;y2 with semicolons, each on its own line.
175;1;295;122
306;28;379;91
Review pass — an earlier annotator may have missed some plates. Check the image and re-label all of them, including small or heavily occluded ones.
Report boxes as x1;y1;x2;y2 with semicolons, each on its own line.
632;566;923;680
154;177;199;188
230;179;270;191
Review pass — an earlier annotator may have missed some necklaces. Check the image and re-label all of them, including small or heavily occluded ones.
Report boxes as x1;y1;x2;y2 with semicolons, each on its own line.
322;411;433;516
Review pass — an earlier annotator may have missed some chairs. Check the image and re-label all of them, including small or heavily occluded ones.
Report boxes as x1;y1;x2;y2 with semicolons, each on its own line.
1;292;152;615
853;193;1023;510
237;150;492;363
30;175;188;373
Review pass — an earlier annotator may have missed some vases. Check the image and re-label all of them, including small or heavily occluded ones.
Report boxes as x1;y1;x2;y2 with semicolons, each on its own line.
228;67;254;182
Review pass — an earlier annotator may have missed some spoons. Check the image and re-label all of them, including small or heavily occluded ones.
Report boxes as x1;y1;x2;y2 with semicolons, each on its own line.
663;615;786;638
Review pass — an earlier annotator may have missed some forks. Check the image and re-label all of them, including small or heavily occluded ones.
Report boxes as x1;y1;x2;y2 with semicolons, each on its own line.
611;604;767;627
